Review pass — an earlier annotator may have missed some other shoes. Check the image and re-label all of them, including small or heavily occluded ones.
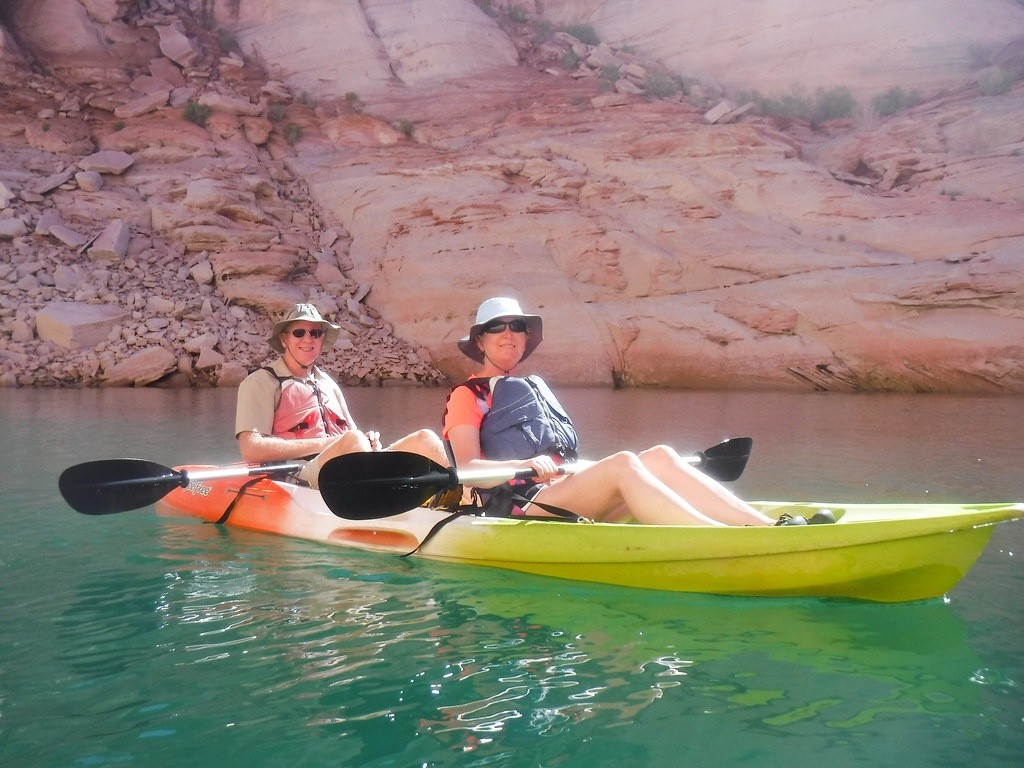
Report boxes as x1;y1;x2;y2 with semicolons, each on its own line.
774;513;807;525
807;509;836;524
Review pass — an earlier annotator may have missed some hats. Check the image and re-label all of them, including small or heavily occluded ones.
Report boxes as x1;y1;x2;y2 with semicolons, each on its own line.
457;297;544;364
265;303;341;354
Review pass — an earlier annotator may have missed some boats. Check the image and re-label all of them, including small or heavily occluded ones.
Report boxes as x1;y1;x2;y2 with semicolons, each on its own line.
158;462;1023;602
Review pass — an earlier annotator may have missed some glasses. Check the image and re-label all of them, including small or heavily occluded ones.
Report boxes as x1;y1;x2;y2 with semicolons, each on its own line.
480;320;527;335
285;328;322;338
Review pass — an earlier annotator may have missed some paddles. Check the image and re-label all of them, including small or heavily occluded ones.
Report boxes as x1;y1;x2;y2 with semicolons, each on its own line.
57;457;306;518
317;435;755;522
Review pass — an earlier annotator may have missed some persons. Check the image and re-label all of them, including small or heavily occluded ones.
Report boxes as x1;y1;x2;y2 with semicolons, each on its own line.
234;304;483;508
442;298;838;527
